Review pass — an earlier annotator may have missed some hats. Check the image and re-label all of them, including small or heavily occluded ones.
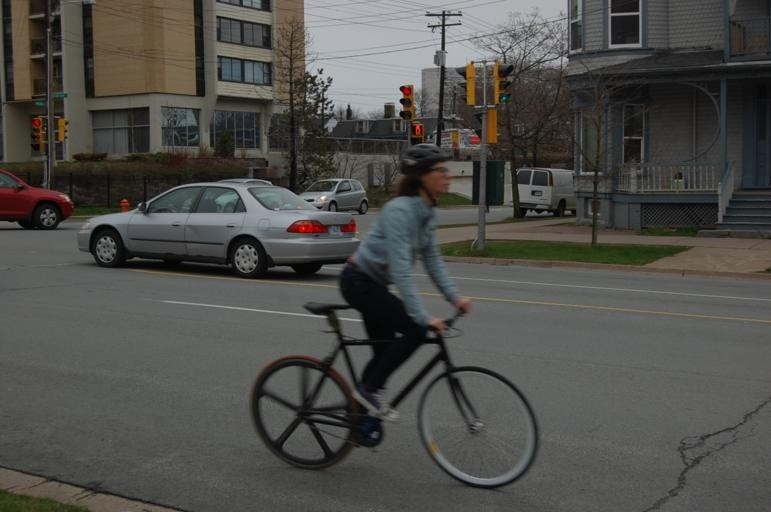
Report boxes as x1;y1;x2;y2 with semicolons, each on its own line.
77;170;369;279
0;170;75;230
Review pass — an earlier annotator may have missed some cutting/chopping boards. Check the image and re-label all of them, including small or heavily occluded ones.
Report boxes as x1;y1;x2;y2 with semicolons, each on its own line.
119;198;130;213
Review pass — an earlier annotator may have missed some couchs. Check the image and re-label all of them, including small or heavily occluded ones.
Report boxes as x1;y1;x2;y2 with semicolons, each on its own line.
455;63;475;107
474;108;498;145
31;115;48;154
399;84;425;138
493;63;514;107
55;118;69;142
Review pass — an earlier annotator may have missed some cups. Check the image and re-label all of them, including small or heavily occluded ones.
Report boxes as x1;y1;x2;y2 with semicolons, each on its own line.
431;165;451;174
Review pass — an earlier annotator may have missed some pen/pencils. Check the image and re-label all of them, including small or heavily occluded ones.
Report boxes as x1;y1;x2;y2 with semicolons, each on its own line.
512;167;576;216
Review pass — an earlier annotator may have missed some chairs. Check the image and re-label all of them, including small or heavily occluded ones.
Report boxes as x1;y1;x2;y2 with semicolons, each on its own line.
352;383;401;424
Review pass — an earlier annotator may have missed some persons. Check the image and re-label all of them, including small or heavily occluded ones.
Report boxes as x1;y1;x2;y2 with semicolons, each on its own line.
335;139;471;428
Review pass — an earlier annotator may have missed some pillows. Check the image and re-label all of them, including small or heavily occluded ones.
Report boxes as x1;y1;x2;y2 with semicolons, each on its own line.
401;142;453;176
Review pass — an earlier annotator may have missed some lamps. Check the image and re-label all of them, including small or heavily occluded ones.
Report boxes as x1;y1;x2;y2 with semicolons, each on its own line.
190;197;245;214
